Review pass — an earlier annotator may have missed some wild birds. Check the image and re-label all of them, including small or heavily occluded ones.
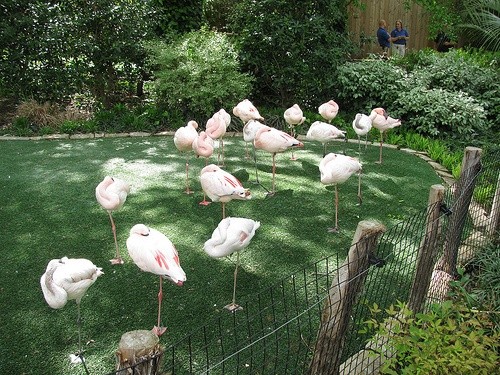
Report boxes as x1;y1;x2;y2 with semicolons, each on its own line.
353;113;373;164
191;130;214;206
318;100;339;124
283;104;306;160
202;216;261;313
233;99;305;197
306;120;347;158
319;153;362;232
200;164;250;219
126;224;187;337
173;120;199;195
39;256;105;358
95;175;129;266
369;107;401;164
205;108;231;166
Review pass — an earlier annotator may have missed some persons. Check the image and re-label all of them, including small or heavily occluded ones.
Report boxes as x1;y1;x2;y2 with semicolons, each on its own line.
390;20;410;58
434;20;458;53
377;20;391;56
